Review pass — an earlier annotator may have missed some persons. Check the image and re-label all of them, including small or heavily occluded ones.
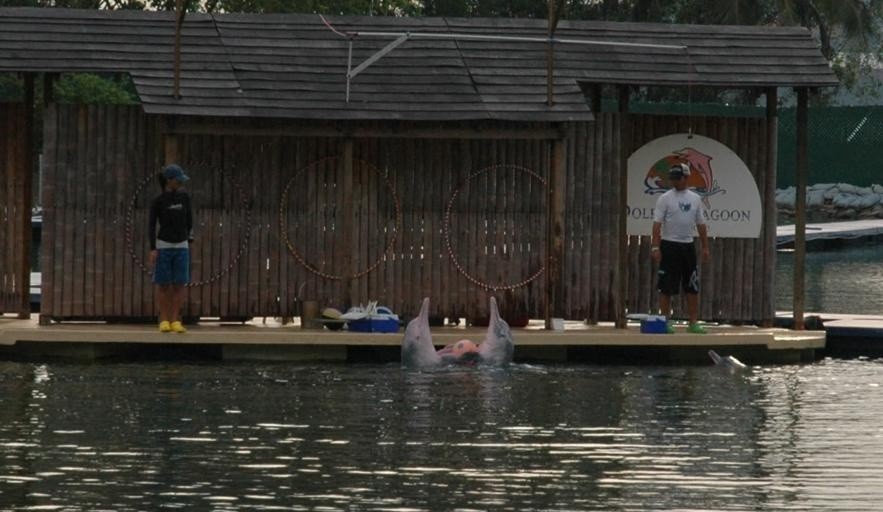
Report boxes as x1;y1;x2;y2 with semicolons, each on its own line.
651;163;711;335
148;161;193;332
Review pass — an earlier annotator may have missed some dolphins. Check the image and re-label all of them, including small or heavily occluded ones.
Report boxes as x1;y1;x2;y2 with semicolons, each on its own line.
708;349;745;369
478;296;513;366
399;297;440;368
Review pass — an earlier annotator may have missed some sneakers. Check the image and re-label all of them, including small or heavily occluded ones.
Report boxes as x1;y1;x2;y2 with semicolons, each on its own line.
685;323;708;334
663;320;675;334
158;319;187;333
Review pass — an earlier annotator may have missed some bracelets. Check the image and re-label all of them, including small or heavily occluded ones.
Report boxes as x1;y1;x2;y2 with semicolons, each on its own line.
651;246;659;252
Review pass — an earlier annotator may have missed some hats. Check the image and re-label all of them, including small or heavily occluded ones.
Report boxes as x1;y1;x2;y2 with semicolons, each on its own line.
160;164;189;183
665;162;691;177
